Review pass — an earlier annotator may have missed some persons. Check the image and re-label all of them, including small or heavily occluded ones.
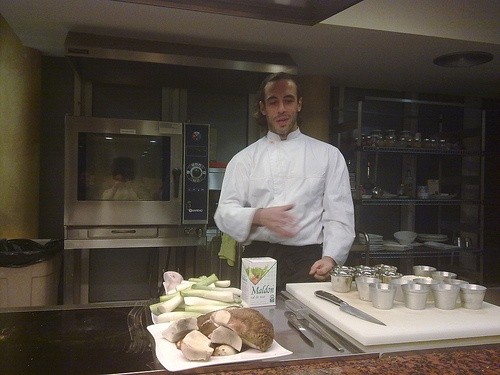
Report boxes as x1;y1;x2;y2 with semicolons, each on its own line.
214;72;356;296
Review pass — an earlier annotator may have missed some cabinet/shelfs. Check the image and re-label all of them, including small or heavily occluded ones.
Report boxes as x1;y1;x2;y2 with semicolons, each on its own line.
329;87;486;287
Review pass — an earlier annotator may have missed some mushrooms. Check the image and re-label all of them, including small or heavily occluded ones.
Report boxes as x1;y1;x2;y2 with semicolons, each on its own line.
162;303;274;360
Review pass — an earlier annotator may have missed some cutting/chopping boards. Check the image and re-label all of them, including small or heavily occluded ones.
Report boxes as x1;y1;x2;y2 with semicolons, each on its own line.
285;281;500;347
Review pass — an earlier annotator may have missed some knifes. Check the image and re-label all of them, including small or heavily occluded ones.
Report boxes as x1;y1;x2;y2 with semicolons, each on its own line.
284;311;313;343
314;290;387;326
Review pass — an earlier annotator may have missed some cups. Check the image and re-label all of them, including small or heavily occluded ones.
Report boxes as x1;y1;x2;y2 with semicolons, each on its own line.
417;186;425;200
427;179;439;195
328;264;488;311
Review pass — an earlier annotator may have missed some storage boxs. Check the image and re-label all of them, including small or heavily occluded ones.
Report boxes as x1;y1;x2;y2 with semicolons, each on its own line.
242;258;277;309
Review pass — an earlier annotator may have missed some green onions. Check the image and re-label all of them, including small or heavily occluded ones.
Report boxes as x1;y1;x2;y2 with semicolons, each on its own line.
150;273;242;323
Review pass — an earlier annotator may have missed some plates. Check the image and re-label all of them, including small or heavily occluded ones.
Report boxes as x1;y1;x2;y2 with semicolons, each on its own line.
384;244;413;252
146;322;293;372
429;194;457;199
354;194;398;199
417;233;449;243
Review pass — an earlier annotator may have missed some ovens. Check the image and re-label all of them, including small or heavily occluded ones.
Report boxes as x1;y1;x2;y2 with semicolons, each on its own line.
65;113;209;300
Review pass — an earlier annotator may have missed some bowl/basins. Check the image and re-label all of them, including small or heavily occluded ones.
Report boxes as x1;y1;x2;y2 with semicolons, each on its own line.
393;231;417;245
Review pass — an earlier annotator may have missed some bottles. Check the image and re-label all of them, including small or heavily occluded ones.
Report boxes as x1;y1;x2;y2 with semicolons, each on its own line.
362;129;447;151
399;184;405;196
